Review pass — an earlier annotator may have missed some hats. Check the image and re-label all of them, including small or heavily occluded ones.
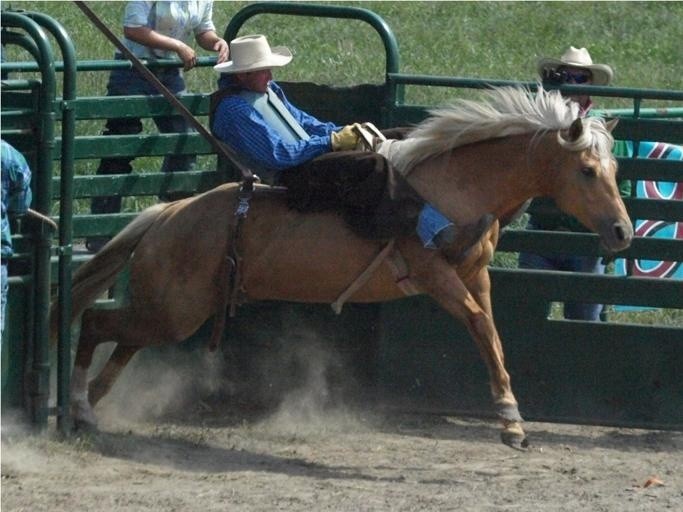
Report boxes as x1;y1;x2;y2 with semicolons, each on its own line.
213;33;293;72
537;46;614;86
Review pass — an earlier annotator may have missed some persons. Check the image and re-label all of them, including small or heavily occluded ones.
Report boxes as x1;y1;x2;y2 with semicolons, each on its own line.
208;31;496;268
0;135;32;367
513;43;615;325
82;0;231;256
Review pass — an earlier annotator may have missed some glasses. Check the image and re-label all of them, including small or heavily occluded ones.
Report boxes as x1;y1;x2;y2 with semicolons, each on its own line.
560;70;592;84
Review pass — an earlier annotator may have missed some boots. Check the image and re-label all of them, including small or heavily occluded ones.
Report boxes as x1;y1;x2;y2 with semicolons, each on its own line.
432;214;494;265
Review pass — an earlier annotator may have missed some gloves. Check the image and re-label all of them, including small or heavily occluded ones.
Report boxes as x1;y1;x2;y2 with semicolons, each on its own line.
331;122;363;152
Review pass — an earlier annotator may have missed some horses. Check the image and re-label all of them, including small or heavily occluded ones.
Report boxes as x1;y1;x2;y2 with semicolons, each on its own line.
47;78;636;455
